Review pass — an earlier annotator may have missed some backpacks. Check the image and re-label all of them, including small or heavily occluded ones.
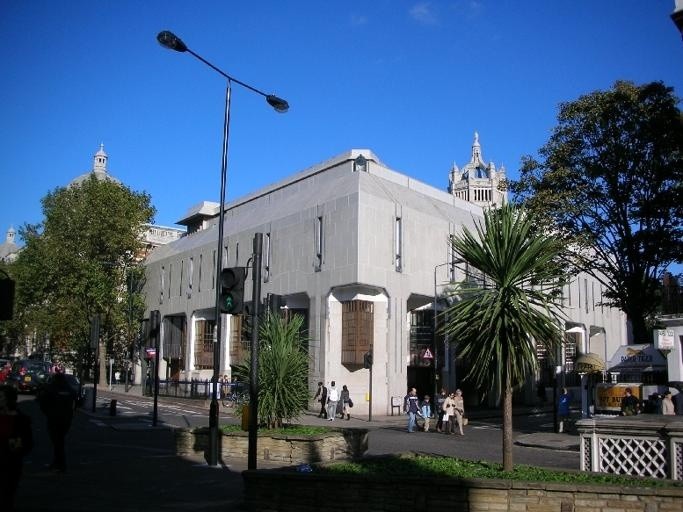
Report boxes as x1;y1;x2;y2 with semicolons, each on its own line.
330;389;339;401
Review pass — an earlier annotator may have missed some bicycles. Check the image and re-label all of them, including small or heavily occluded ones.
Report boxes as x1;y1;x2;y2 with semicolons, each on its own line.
220;382;249;409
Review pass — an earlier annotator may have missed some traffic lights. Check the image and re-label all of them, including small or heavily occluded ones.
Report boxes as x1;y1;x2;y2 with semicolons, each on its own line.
269;294;286;321
218;265;245;316
244;301;267;344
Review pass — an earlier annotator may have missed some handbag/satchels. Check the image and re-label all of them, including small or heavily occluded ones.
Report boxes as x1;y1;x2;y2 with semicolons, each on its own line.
349;399;353;407
442;413;449;422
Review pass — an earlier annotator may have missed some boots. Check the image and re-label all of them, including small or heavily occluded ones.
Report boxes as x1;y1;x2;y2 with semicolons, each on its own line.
346;414;350;420
341;414;345;419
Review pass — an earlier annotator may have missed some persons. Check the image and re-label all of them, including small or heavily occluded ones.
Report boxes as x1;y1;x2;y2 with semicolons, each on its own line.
402;387;417;432
339;385;350;420
311;381;326;419
453;388;465;436
432;388;447;433
442;391;457;436
618;387;639;415
218;374;238;397
661;390;676;415
115;370;121;387
325;380;338;421
418;395;431;432
556;387;570;432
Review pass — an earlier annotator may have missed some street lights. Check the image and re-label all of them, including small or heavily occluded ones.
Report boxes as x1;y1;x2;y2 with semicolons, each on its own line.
155;28;292;466
432;257;466;417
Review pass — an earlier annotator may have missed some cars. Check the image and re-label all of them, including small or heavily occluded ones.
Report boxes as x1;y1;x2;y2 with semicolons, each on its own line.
0;348;87;399
